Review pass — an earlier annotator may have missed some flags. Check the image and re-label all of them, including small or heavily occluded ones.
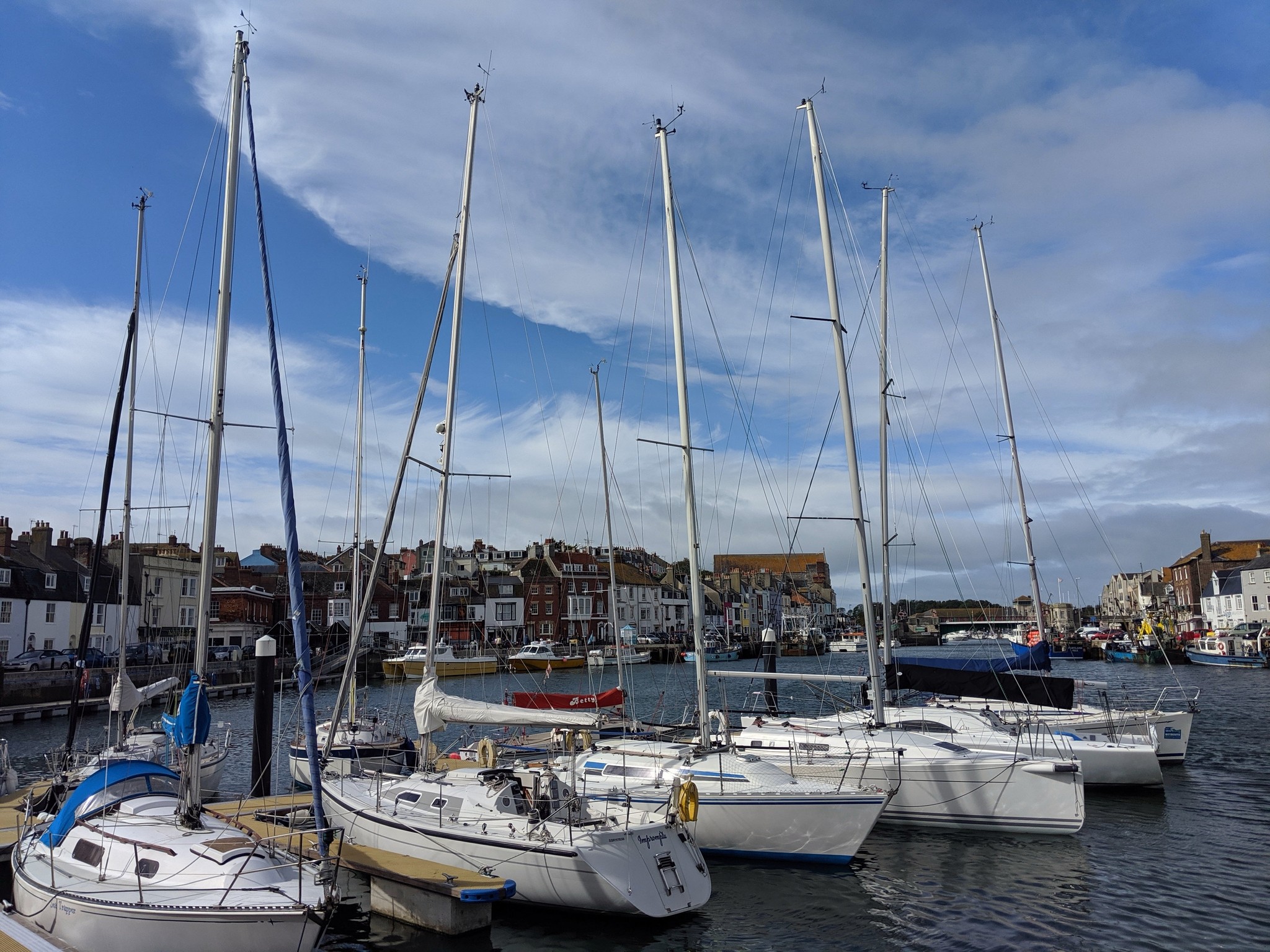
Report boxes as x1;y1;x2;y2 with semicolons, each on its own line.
587;633;594;645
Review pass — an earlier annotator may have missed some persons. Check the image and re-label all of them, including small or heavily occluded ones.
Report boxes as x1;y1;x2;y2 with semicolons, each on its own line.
494;635;502;649
558;634;567;647
276;642;297;658
575;632;583;646
505;636;511;649
1110;640;1116;652
521;634;529;647
488;635;492;649
328;641;333;647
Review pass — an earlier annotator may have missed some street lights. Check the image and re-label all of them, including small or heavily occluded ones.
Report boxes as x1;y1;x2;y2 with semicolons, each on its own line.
365;607;374;648
1098;594;1103;622
1076;578;1081;627
874;605;877;623
660;601;665;633
739;603;744;633
528;604;533;644
145;588;155;666
574;607;579;640
849;605;852;618
764;612;767;628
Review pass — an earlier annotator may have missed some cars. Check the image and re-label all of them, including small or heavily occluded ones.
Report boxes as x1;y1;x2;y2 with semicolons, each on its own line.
2;649;71;672
1111;630;1140;641
1091;629;1125;641
636;634;661;644
61;646;112;670
1079;631;1101;638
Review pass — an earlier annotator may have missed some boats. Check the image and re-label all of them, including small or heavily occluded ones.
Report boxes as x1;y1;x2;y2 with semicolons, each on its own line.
1182;627;1270;668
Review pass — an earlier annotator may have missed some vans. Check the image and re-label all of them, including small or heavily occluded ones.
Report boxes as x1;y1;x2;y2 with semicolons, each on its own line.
1227;622;1262;637
826;632;834;636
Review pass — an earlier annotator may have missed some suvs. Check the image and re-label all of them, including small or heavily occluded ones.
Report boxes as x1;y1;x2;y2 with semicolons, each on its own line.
647;631;675;644
703;631;724;643
167;641;216;665
241;645;256;659
1176;629;1213;641
109;643;163;666
1074;627;1102;638
670;631;693;644
214;645;243;661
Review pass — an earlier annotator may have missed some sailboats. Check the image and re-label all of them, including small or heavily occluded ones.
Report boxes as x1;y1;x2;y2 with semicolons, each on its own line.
11;10;1202;952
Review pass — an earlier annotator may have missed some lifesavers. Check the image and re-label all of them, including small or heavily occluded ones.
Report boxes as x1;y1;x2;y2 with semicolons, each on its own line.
678;781;698;822
611;646;616;648
621;645;631;647
1218;643;1224;650
566;728;592;753
708;711;725;733
477;738;497;768
